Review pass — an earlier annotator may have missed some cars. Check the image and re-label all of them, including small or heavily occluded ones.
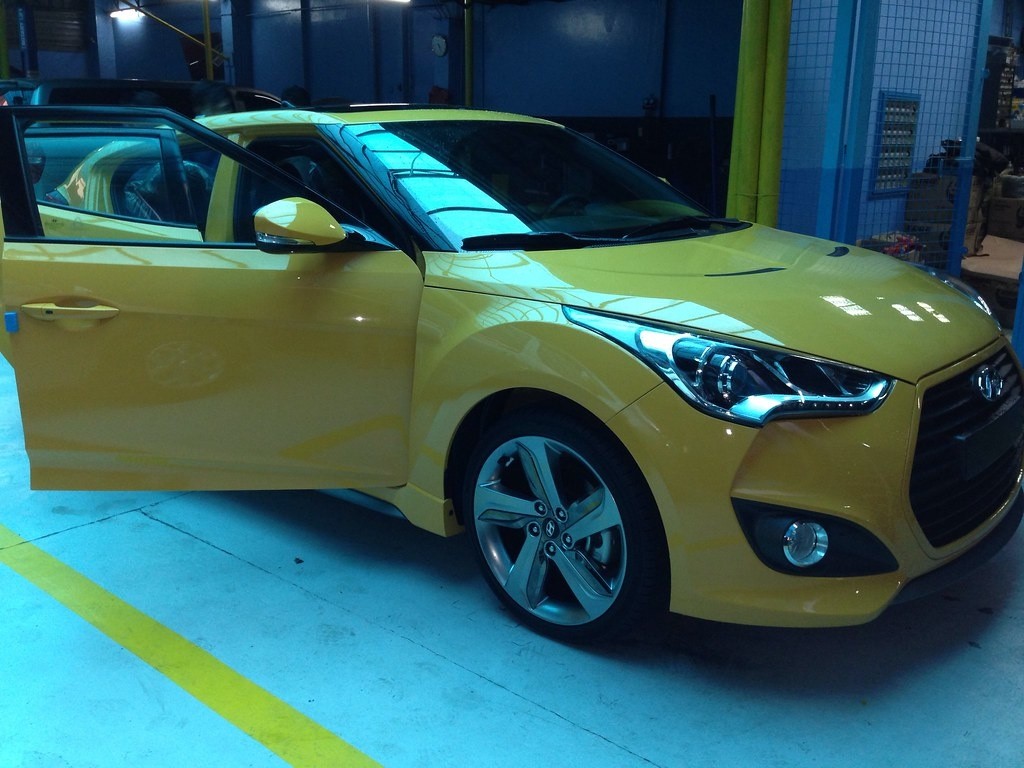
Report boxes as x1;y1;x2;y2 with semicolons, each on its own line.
0;95;1022;652
0;80;285;122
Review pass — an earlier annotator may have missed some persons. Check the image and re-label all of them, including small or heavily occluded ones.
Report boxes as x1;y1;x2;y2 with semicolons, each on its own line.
190;79;244;116
428;86;453;103
281;84;311;107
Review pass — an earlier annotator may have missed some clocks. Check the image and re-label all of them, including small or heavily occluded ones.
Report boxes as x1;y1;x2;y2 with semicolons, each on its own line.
429;33;447;58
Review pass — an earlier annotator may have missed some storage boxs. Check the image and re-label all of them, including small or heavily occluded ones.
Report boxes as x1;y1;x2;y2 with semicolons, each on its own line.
981;34;1024;130
850;163;1024;269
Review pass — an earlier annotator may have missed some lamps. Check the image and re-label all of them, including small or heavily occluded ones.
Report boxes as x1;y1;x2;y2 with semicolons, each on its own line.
641;97;658;110
107;3;149;18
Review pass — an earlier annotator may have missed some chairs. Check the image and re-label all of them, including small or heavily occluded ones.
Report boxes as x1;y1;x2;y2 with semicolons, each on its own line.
258;159;367;230
140;158;215;236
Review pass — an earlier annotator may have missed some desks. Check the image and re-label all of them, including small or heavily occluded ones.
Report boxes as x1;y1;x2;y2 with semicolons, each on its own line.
978;127;1024;163
